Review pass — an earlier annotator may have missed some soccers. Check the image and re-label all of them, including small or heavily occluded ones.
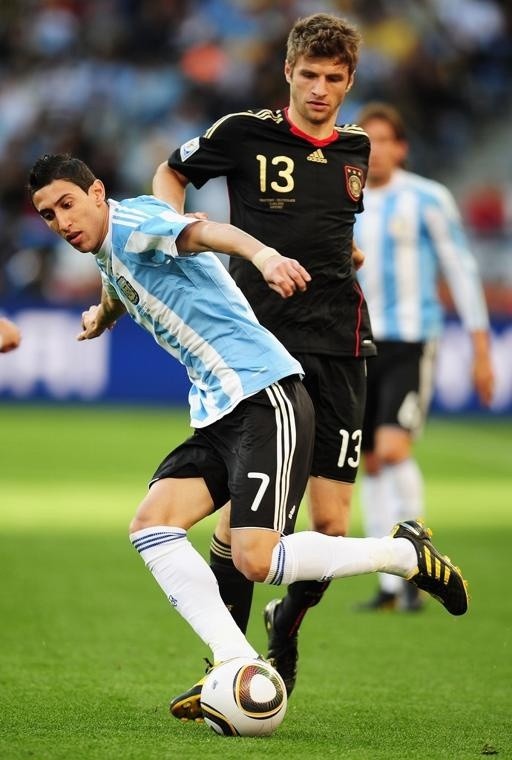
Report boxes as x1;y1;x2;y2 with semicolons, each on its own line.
199;657;288;737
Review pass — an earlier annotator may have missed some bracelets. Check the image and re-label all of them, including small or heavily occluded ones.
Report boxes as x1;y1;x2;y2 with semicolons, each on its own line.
251;246;280;272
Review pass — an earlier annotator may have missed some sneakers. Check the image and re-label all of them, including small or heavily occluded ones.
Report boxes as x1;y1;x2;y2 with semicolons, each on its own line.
350;579;427;614
167;650;277;724
389;516;470;617
262;598;301;704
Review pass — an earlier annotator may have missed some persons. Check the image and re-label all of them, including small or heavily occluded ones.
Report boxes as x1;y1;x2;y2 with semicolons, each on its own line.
152;12;379;726
25;152;466;726
344;102;496;611
